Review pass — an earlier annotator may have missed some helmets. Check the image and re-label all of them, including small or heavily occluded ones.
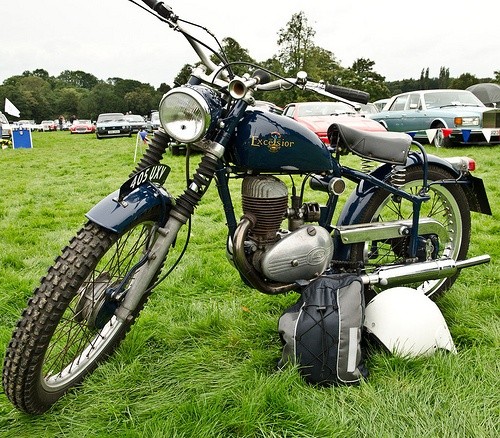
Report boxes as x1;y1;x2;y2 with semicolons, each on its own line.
361;286;458;363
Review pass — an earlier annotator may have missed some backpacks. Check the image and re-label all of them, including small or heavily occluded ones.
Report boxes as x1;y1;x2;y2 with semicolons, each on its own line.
278;275;370;387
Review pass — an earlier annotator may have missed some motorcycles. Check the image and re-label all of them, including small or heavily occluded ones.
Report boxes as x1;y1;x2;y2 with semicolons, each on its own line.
2;0;492;415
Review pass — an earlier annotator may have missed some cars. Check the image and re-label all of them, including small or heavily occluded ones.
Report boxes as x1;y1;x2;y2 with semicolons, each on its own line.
280;88;500;148
0;112;162;139
